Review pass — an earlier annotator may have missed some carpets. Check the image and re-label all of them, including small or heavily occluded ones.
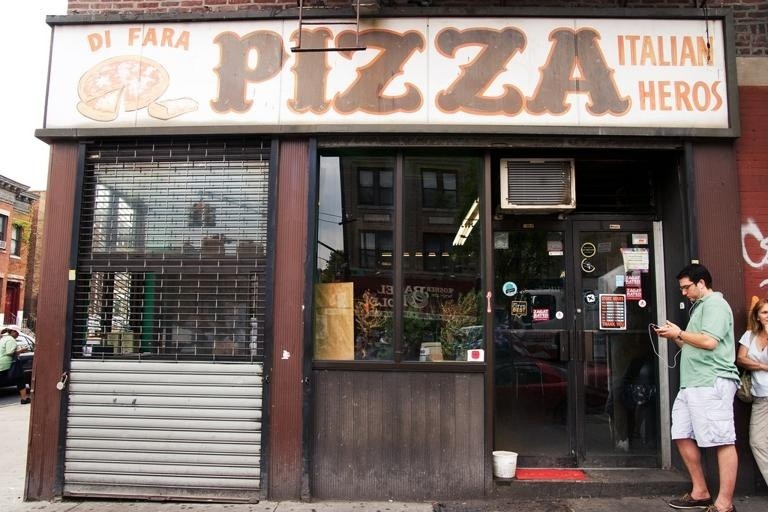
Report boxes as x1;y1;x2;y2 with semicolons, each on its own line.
516;468;589;480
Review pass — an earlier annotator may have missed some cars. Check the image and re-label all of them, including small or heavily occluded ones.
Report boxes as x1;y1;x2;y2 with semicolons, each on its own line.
2;334;36;389
496;362;609;425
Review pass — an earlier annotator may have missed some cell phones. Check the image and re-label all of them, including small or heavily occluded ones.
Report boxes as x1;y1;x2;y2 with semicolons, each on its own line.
653;326;660;329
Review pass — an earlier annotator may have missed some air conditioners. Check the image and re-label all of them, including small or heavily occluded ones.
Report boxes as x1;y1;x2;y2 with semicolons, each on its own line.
500;157;577;211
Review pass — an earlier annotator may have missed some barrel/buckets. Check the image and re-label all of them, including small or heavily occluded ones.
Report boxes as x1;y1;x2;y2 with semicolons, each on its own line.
492;451;519;478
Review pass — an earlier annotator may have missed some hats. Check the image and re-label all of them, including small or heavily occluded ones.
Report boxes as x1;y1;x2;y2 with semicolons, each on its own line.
8;325;20;335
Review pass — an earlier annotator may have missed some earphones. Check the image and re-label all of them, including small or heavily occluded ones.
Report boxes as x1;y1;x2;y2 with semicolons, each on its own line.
700;283;702;287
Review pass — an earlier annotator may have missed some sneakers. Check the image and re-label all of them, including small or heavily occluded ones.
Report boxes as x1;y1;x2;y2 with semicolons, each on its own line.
21;398;31;404
668;493;736;512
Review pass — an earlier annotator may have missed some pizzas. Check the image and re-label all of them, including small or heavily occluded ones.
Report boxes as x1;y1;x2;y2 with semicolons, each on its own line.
75;55;169;122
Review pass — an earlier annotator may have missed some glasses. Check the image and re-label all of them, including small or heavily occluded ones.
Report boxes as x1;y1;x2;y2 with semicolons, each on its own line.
680;283;694;290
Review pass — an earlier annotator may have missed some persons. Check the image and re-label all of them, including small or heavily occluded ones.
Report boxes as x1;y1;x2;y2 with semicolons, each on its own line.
0;325;32;404
736;297;768;485
654;264;744;511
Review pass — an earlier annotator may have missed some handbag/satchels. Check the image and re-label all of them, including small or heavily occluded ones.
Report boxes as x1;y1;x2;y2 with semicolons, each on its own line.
8;353;25;381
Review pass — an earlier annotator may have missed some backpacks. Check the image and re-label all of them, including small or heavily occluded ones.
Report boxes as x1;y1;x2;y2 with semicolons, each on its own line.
736;370;753;402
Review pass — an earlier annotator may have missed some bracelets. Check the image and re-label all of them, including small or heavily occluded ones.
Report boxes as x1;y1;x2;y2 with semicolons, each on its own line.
677;329;685;340
